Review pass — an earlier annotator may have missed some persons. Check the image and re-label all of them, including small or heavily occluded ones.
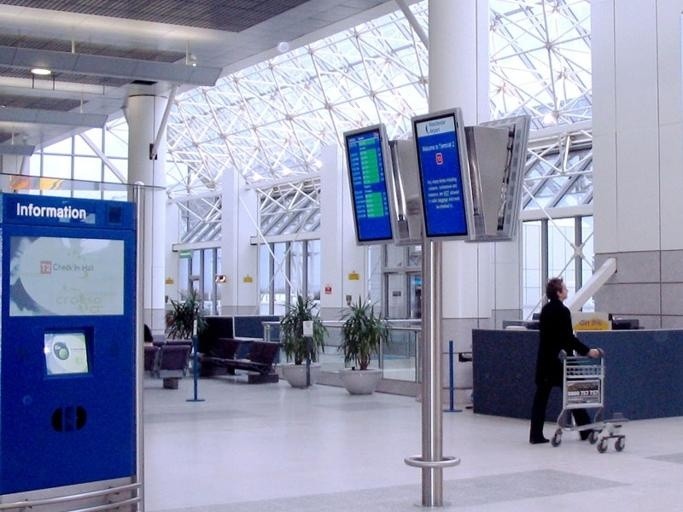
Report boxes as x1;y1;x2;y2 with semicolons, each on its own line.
530;278;601;444
10;236;41;316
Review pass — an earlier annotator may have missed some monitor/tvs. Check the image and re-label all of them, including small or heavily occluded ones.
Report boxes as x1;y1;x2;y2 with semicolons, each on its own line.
344;123;400;246
469;114;533;243
408;107;476;244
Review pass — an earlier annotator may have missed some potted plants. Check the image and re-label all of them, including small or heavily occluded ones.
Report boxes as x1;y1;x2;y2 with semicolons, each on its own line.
278;289;395;394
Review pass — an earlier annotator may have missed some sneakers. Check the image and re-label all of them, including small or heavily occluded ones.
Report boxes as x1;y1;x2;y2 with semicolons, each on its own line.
580;430;594;440
531;437;549;443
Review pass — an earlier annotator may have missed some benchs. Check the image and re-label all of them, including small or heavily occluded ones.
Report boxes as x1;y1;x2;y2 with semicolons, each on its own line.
142;338;283;389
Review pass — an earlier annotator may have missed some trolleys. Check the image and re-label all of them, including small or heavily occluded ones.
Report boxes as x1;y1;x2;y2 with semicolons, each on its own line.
550;346;628;453
